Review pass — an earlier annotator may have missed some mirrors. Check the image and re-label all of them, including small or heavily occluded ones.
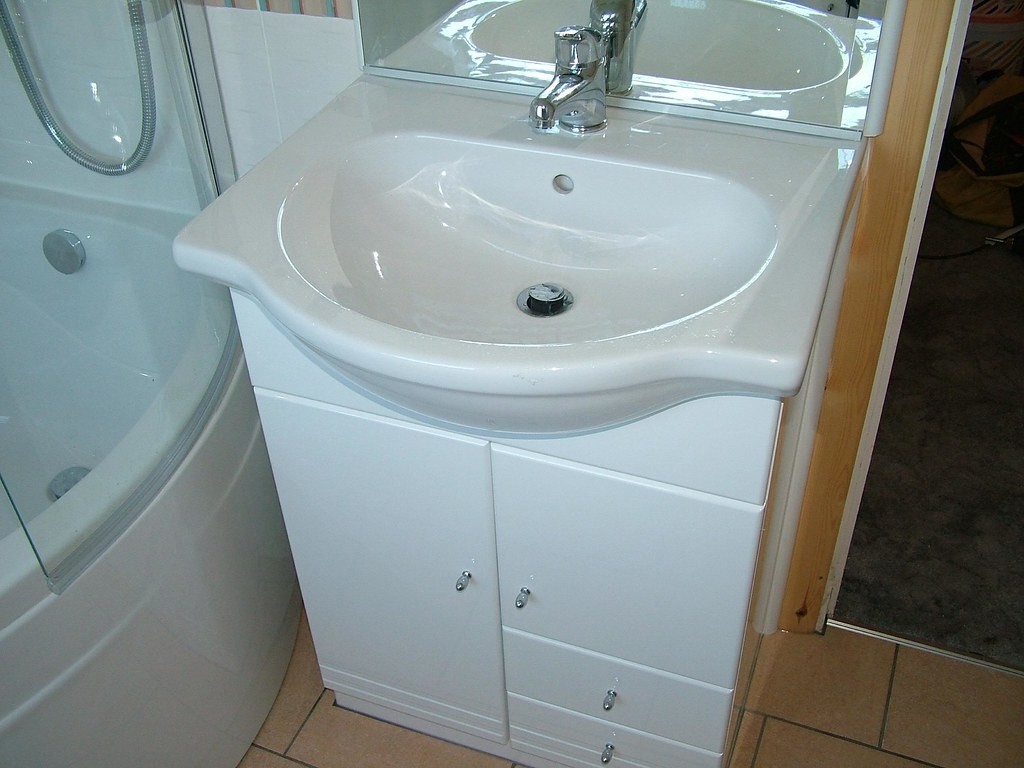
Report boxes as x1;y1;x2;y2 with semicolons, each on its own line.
351;0;887;144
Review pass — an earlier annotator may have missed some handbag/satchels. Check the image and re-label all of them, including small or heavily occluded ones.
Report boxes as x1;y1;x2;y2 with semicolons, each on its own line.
932;76;1024;246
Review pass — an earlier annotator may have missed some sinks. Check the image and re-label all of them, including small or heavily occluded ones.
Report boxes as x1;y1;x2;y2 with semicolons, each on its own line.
369;1;884;130
167;73;861;438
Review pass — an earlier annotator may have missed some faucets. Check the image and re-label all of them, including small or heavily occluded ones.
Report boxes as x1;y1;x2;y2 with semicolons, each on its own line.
530;27;608;131
589;0;653;92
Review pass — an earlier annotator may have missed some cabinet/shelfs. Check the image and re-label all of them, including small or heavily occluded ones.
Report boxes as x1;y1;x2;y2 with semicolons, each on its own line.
228;286;783;768
